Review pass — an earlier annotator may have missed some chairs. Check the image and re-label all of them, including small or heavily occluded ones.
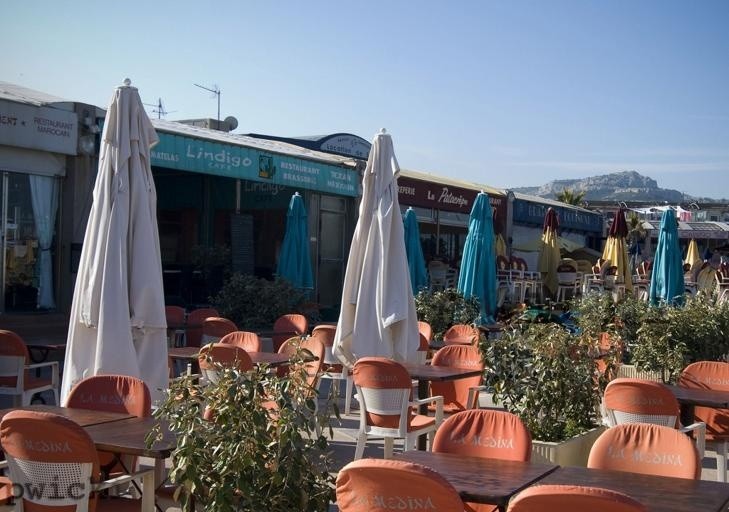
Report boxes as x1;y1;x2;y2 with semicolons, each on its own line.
425;256;729;303
0;304;729;512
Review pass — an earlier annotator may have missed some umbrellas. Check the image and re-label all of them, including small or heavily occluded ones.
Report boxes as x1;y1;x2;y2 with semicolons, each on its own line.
275;190;315;300
403;205;430;296
496;231;506;256
648;209;686;307
58;74;171;409
602;207;634;294
330;125;423;369
537;206;562;273
685;236;701;270
457;187;497;323
